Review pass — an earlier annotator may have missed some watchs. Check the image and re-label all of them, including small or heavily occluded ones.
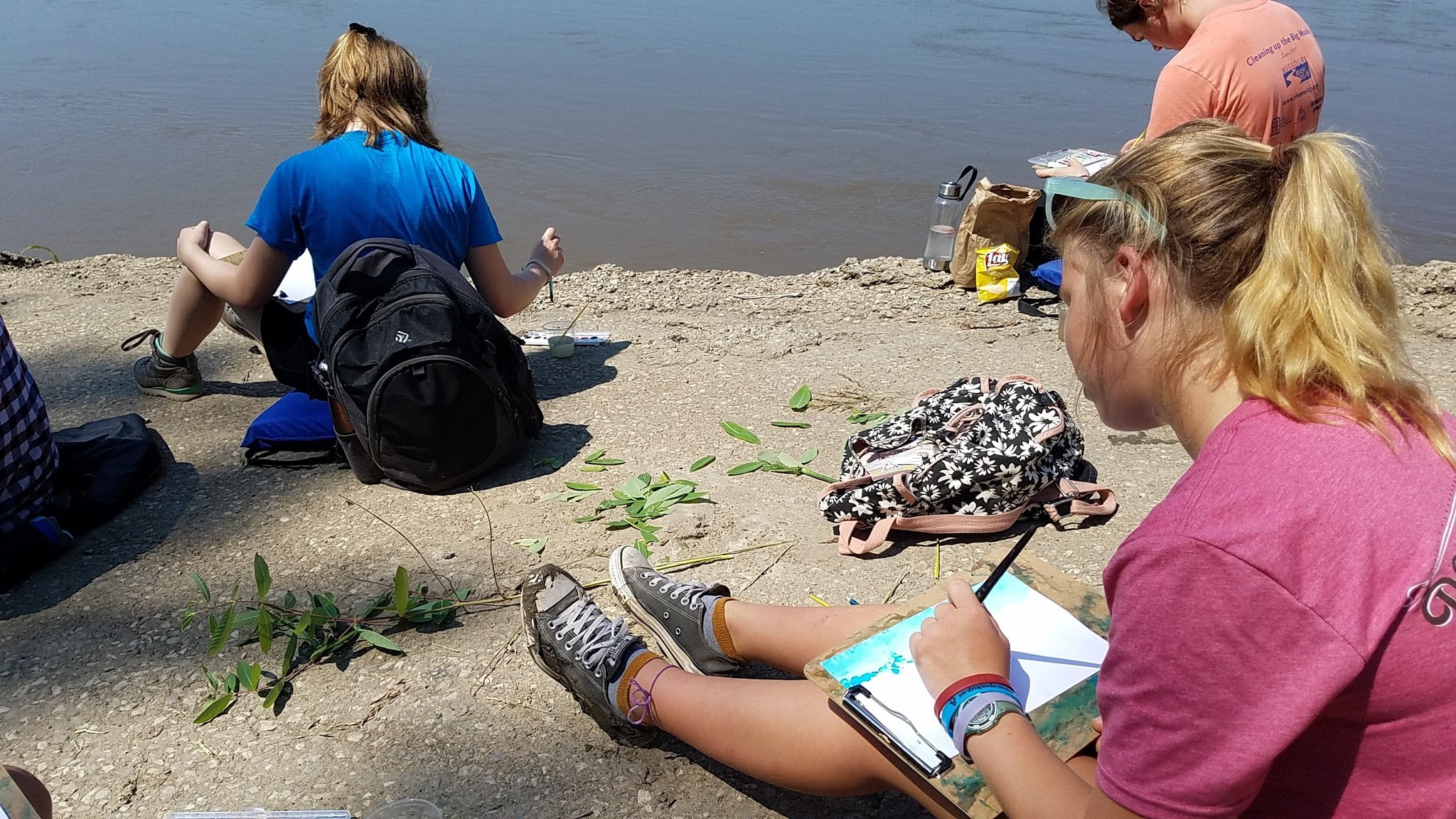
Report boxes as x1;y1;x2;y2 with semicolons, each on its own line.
949;692;1030;741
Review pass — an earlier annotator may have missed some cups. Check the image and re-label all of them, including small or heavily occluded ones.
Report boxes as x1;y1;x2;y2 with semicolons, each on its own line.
542;321;577;358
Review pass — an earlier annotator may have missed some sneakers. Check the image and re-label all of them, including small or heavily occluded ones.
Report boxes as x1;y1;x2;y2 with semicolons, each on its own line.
121;329;206;401
521;564;658;747
608;546;748;679
220;301;258;342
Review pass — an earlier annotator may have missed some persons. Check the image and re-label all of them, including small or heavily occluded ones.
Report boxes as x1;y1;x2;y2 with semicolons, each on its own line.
0;314;60;536
119;23;564;396
1029;0;1325;262
522;114;1455;819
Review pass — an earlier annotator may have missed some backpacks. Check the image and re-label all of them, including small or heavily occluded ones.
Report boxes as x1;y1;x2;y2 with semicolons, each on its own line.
818;375;1115;554
308;239;544;492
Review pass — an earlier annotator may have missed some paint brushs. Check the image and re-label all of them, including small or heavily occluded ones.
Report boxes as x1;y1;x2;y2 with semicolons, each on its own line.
550;277;553;302
975;525;1037;604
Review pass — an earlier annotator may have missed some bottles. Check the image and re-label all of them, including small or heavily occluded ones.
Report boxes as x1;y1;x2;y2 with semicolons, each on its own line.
922;180;962;271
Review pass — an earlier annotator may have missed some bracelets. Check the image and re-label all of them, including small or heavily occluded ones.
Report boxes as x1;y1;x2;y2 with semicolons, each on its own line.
934;674;1023;738
522;260;554;302
953;691;1022;761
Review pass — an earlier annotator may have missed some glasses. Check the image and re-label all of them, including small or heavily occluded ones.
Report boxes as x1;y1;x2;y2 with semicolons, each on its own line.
1044;175;1168;242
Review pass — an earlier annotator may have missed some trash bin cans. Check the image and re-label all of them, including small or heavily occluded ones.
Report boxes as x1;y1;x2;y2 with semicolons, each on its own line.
1130;127;1147;149
